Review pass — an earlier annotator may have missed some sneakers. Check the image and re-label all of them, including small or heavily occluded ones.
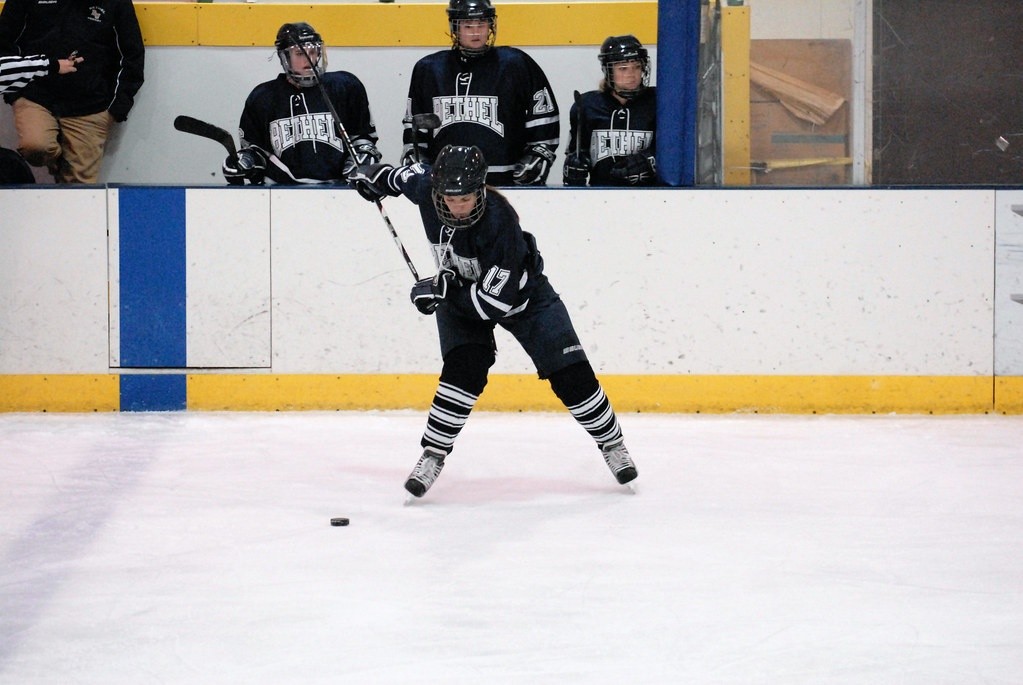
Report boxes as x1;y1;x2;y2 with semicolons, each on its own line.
601;435;638;492
404;446;448;505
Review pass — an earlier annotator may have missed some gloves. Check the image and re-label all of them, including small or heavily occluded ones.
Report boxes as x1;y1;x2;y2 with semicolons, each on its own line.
513;144;557;186
563;151;592;186
410;272;457;315
355;163;407;200
223;146;268;187
610;150;657;185
342;145;382;188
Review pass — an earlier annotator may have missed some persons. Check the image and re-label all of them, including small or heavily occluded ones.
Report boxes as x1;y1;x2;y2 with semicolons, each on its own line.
347;144;641;499
238;22;382;186
399;0;560;186
0;0;145;184
562;35;660;187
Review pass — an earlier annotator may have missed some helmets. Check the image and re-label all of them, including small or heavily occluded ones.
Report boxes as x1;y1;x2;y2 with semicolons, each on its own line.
275;22;328;88
598;35;650;100
431;145;489;232
446;0;497;59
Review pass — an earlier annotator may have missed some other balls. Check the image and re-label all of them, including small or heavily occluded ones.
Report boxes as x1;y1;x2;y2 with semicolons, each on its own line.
330;518;350;526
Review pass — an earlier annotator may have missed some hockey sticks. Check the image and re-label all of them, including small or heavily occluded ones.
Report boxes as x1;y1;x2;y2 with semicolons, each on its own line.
289;23;441;313
568;86;585;186
407;109;440;163
171;114;241;184
